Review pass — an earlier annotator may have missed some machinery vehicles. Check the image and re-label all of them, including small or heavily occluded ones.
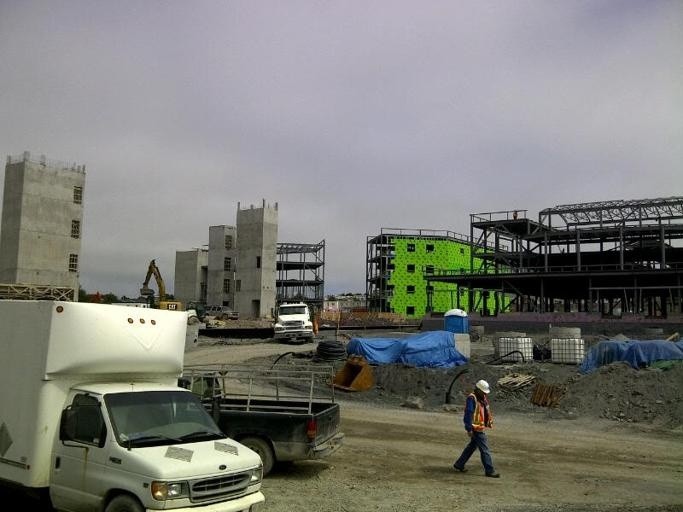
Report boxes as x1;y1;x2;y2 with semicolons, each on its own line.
140;260;182;311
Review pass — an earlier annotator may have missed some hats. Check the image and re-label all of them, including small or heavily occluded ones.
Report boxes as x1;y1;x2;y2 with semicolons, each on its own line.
473;379;491;394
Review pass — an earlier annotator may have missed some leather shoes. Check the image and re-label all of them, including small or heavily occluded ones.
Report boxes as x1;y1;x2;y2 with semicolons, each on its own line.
453;463;467;472
485;470;499;478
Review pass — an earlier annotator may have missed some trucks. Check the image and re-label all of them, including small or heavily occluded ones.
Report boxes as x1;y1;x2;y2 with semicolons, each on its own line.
0;298;267;512
272;302;315;345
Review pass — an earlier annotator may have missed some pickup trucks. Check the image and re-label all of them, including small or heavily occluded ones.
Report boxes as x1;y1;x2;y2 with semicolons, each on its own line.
177;364;344;475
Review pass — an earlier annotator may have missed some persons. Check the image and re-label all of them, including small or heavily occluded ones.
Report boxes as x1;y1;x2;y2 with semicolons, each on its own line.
451;379;500;478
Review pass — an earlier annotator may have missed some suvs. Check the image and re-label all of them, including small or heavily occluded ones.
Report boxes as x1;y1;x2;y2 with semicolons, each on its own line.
205;305;238;320
